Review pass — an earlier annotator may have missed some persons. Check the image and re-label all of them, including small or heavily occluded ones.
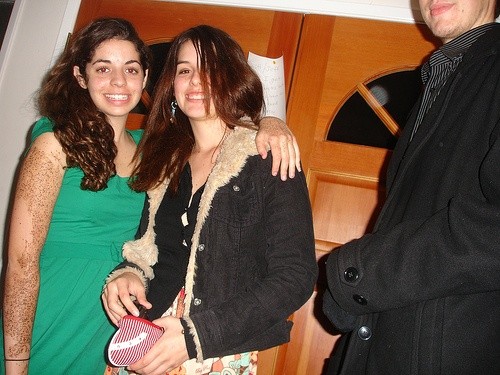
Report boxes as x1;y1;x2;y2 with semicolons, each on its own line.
311;1;500;375
100;25;318;374
0;16;305;375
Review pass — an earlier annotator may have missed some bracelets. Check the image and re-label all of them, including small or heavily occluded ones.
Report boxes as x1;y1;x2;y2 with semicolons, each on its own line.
4;357;31;362
